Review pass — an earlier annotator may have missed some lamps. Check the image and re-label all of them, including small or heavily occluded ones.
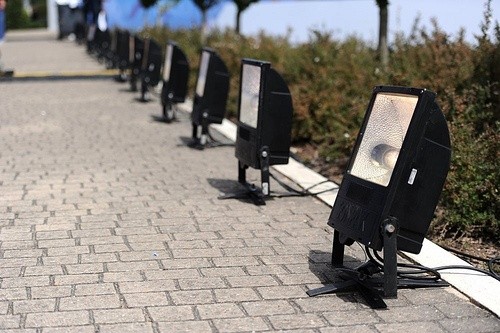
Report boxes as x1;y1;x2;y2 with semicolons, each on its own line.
151;39;189;124
85;24;146;93
175;47;230;149
216;58;294;205
306;85;453;298
133;36;162;104
